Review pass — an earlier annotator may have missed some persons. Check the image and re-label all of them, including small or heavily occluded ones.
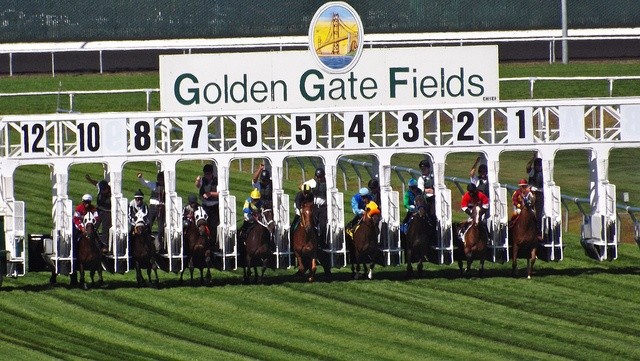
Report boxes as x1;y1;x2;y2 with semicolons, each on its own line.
181;194;210;242
459;183;494;240
416;158;435;212
128;188;151;251
239;188;266;237
136;170;167;254
527;150;546;219
347;188;376;238
401;178;429;234
74;193;101;257
468;154;490;205
84;171;112;254
311;168;327;226
510;177;533;217
194;163;220;252
291;185;318;241
251;164;275;235
365;178;381;226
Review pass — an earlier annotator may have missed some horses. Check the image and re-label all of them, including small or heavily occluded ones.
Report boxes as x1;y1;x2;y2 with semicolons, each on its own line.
128;205;162;289
72;210;102;290
237;205;276;281
508;188;539;279
345;200;381;279
453;198;488;279
183;202;213;286
400;188;430;272
290;202;319;282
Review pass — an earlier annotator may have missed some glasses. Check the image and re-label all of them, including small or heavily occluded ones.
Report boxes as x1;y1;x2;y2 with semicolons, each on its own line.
136;197;143;200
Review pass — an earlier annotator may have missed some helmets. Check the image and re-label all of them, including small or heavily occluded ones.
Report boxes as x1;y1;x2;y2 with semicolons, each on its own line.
250;188;262;199
408;179;418;186
157;172;164;180
134;189;144;198
99;180;108;186
369;179;378;187
518;178;528;186
419;160;430;168
203;164;213;173
188;195;197;202
478;164;487;173
314;168;325;177
82;193;93;201
467;183;476;191
261;170;270;179
359;186;369;195
